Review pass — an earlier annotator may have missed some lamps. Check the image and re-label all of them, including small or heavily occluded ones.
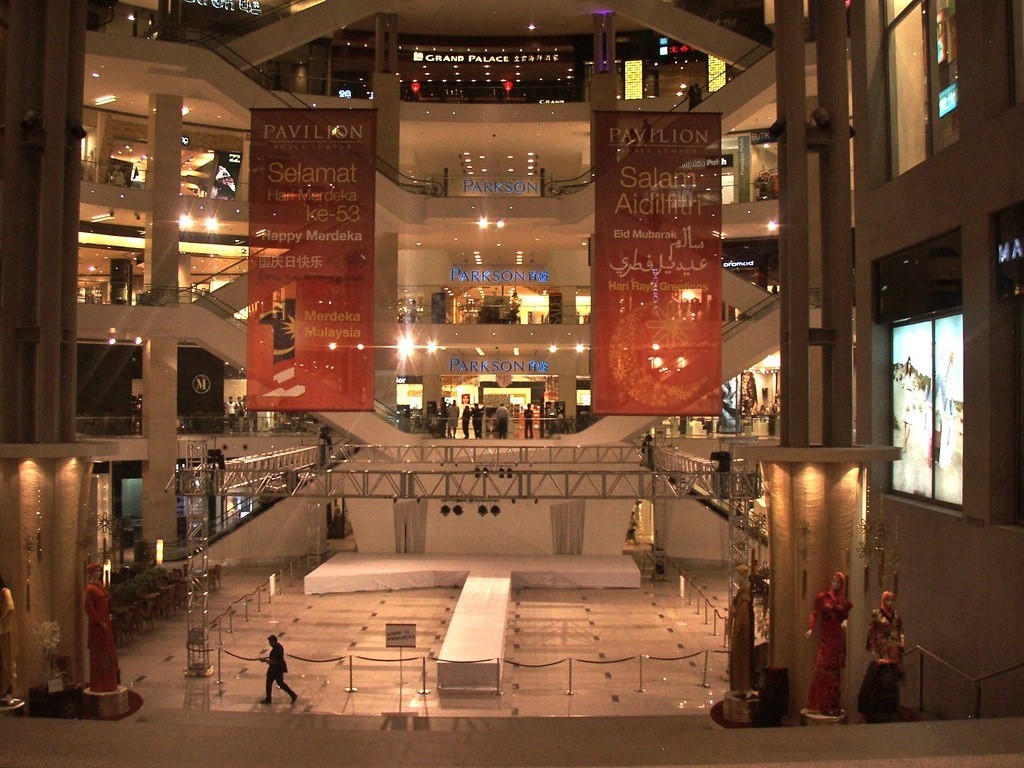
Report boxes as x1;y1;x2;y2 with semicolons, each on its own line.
452;502;463;515
507;467;512;478
474;467;481;478
499;467;505;479
439;502;451;516
477;502;489;518
135;210;140;220
490;504;501;517
484;467;489;478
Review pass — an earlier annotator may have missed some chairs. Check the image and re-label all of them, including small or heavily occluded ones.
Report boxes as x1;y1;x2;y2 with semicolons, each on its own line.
110;563;223;647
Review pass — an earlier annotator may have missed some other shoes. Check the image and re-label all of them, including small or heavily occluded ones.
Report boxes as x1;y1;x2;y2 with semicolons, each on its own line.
261;700;272;704
290;695;298;704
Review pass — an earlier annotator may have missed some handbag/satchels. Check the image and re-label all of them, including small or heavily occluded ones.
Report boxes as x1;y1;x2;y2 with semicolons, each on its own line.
282;660;288;672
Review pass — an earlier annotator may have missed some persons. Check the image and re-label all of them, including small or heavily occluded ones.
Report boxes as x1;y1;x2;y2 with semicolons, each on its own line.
471;404;482;438
760;405;769;414
462;406;471;439
84;563;120;691
447;400;459;439
686;84;702;108
226;396;247;427
626;120;652;151
261;635;298;705
858;591;904;723
510;404;520;415
626;512;638;543
524;404;533;438
0;575;15;705
113;169;122;184
410;300;417;322
729;565;754;691
641;436;652;453
804;573;853;716
496;404;508;438
